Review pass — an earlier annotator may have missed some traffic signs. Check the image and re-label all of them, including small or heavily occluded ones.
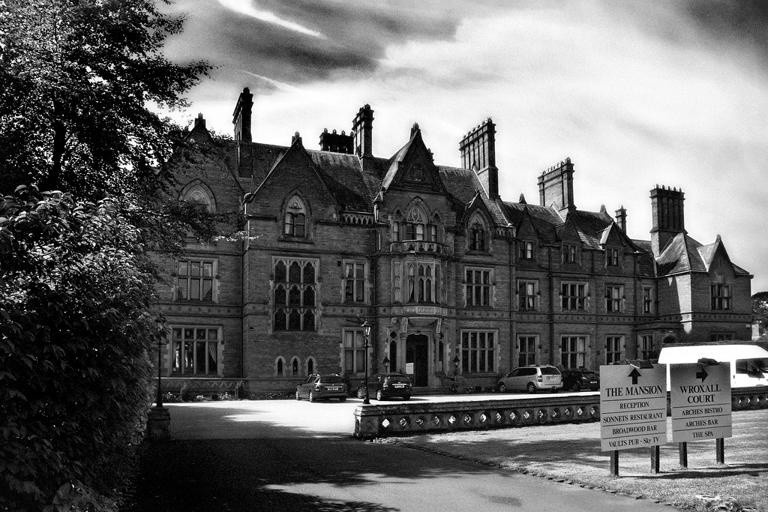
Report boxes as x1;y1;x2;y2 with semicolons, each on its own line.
670;362;732;442
599;365;667;453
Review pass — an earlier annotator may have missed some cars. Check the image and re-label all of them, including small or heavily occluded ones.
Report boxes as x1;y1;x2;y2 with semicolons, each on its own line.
357;373;412;401
497;364;599;394
296;373;346;402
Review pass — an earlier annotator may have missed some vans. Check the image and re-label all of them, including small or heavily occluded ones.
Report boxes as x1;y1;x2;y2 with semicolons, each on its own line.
657;344;768;391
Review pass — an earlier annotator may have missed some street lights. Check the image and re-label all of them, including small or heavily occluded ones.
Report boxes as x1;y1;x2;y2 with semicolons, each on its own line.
154;314;168;407
361;319;373;404
